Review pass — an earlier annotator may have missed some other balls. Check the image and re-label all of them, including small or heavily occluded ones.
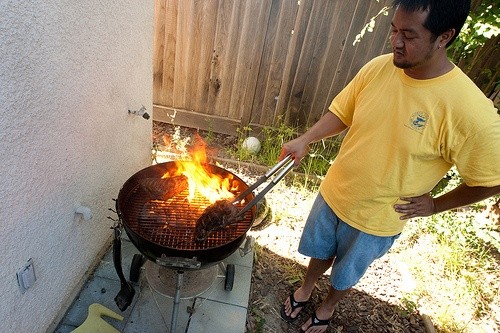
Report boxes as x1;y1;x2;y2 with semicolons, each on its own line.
243;137;261;154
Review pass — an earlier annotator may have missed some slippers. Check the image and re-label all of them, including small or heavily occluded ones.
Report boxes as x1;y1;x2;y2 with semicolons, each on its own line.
280;292;312;322
300;308;335;332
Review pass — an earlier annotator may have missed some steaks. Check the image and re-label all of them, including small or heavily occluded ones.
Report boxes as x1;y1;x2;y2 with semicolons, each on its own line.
138;175;188;200
194;200;247;242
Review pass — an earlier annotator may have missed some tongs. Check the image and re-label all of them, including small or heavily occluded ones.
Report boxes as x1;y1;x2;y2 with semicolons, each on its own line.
229;151;298;220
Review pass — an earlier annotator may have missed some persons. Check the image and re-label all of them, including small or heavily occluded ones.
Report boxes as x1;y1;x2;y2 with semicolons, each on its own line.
276;0;500;333
